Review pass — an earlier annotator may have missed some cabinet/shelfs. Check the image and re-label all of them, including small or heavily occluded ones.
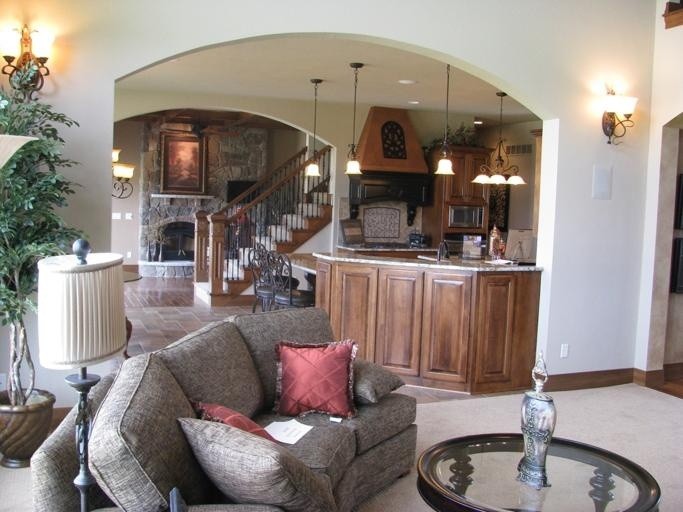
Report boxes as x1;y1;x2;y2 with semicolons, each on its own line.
419;144;497;253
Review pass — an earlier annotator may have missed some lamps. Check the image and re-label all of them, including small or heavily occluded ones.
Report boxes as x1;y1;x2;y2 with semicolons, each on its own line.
600;83;640;147
0;18;53;103
111;145;135;200
432;63;457;175
33;234;129;512
342;62;365;175
469;92;528;186
302;78;322;178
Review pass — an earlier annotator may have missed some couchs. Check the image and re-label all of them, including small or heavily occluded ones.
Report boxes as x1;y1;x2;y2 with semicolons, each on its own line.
27;305;419;511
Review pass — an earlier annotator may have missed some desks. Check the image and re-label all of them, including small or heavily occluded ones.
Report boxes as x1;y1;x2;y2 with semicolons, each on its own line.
274;253;315;291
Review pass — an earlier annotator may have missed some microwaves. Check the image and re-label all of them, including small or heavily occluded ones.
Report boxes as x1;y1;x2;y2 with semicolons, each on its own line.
448;205;483;228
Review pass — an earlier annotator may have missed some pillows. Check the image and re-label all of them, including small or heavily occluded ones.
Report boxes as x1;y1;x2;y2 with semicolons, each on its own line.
175;417;339;511
269;331;364;422
354;360;405;406
188;392;277;444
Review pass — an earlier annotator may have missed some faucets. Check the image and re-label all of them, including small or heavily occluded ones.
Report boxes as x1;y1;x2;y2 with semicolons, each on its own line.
437;239;450;263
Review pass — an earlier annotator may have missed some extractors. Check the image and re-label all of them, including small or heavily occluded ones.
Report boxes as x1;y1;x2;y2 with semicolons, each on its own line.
350;170;434;226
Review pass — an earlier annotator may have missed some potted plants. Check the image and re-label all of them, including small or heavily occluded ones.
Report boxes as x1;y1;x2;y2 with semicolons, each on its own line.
0;82;90;471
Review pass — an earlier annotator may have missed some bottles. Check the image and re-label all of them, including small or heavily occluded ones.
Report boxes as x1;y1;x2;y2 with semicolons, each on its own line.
489;224;504;260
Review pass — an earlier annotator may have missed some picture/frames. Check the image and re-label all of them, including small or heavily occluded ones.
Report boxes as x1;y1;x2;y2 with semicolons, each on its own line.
158;131;209;195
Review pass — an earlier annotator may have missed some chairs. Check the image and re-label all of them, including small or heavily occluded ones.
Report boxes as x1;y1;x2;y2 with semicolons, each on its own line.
247;248;274;314
265;249;315;314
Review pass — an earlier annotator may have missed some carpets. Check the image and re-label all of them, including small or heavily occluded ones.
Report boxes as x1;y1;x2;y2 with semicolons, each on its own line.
0;380;682;511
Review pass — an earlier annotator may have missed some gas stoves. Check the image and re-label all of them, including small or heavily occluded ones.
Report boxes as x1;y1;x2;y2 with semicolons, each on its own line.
335;241;413;252
